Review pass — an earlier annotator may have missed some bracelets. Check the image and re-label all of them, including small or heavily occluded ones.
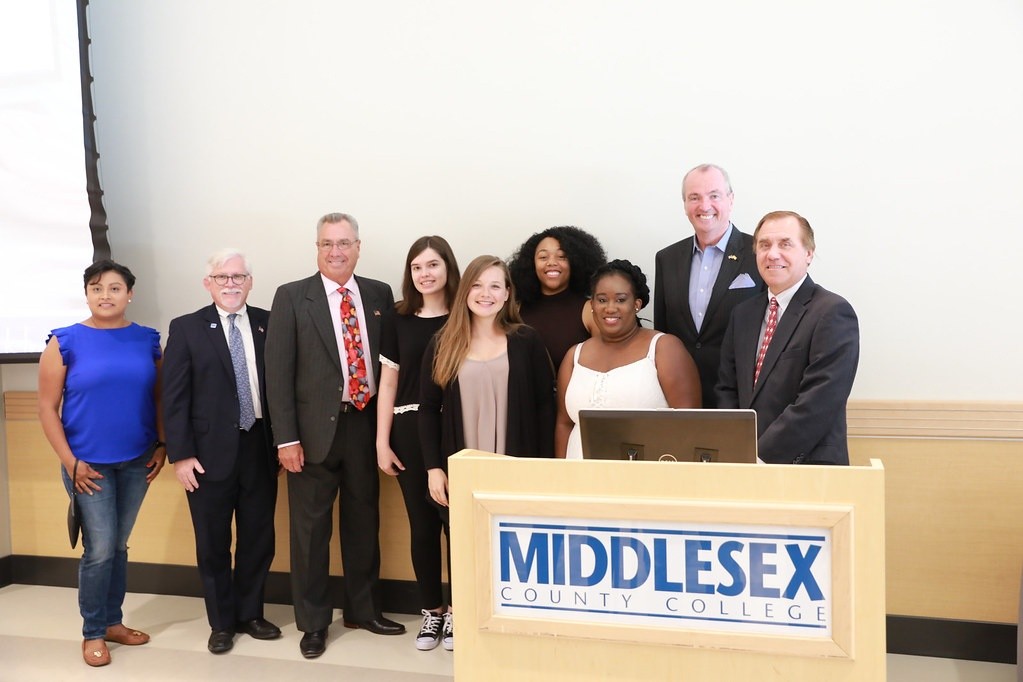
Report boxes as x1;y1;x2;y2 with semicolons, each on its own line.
156;441;165;449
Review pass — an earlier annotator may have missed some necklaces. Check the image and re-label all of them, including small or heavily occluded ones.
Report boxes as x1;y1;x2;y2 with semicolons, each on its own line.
90;318;123;329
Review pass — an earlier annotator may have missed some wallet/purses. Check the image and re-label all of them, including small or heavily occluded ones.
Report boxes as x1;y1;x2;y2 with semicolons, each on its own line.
67;459;79;550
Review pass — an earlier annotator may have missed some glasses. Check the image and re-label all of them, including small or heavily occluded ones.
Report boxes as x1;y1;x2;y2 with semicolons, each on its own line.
316;241;356;250
209;274;249;286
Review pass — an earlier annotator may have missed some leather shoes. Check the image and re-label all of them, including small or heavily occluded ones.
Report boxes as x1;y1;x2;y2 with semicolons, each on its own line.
208;629;235;652
299;626;329;658
343;608;405;635
236;619;281;640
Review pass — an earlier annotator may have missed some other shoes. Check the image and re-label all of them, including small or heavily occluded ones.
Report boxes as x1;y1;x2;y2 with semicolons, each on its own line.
82;639;111;667
104;624;150;645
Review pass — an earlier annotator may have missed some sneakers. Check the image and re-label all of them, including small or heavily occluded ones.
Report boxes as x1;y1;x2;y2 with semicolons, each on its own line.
415;609;442;651
442;612;453;651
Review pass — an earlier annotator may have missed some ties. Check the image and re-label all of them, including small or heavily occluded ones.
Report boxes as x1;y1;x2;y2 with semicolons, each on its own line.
337;288;370;411
753;297;777;393
228;314;256;432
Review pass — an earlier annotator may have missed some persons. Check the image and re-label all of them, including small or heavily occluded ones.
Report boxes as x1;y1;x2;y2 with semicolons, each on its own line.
653;163;768;408
161;250;286;653
36;259;166;667
418;225;704;512
375;236;463;650
708;211;860;466
264;213;405;659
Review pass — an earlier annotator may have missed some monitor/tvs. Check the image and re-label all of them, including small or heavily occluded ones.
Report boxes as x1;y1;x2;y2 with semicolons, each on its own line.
578;409;758;464
